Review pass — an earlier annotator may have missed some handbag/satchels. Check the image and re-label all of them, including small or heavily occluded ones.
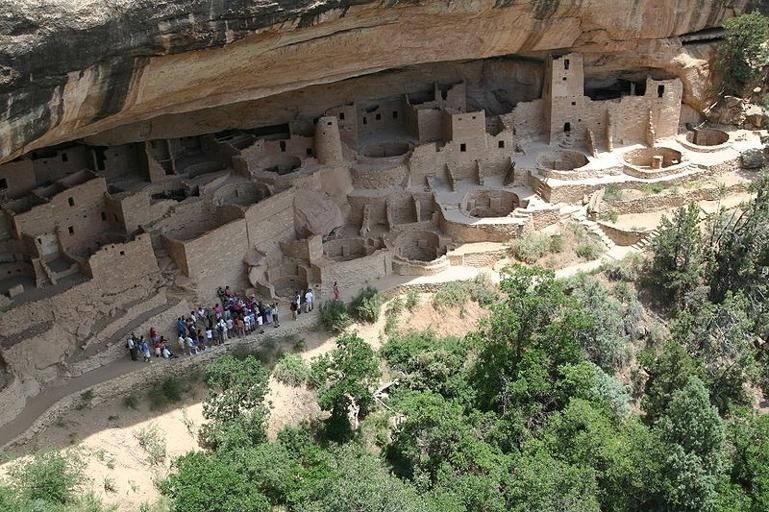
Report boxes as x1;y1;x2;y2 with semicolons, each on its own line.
125;344;129;348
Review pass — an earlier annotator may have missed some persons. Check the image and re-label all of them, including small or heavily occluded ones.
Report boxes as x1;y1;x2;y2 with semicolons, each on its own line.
333;281;340;301
124;284;315;364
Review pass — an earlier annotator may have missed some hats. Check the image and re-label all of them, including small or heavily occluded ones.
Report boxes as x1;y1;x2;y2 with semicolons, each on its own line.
127;335;133;339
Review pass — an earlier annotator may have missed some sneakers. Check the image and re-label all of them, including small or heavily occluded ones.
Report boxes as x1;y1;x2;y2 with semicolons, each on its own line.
214;321;280;346
148;361;153;363
144;359;148;362
